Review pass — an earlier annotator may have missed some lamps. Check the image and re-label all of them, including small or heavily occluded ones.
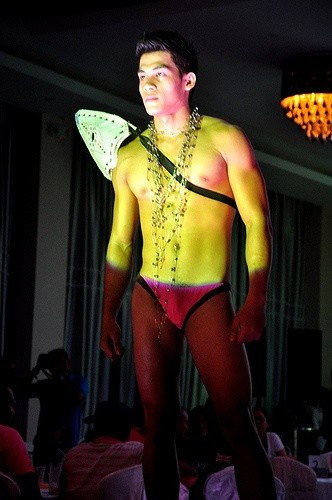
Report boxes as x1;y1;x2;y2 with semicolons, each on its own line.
279;68;332;142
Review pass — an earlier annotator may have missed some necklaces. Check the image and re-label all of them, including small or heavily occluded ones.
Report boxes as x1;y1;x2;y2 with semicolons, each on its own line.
147;107;201;340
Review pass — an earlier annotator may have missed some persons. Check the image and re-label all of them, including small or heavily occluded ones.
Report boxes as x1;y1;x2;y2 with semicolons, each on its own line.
56;402;287;500
17;349;89;483
0;383;42;500
305;387;332;454
98;30;274;500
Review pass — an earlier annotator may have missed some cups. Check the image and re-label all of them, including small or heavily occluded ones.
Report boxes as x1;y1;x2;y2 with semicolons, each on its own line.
37;467;45;484
49;462;63;493
